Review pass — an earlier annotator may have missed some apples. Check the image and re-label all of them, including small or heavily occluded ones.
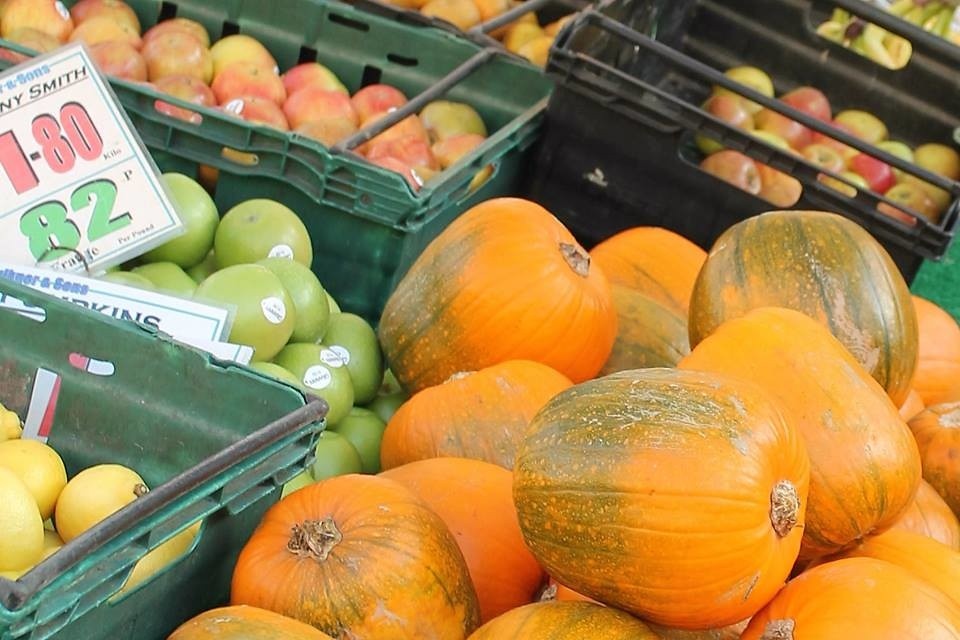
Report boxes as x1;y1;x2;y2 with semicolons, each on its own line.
0;0;588;195
96;174;408;501
683;63;958;243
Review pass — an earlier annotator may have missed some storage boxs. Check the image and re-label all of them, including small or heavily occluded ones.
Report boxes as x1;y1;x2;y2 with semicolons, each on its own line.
4;4;960;637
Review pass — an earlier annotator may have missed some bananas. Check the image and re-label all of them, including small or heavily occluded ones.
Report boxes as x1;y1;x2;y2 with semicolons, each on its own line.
817;0;960;71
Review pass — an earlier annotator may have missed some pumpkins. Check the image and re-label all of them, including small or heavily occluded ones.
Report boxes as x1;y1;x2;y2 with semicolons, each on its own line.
171;195;960;640
